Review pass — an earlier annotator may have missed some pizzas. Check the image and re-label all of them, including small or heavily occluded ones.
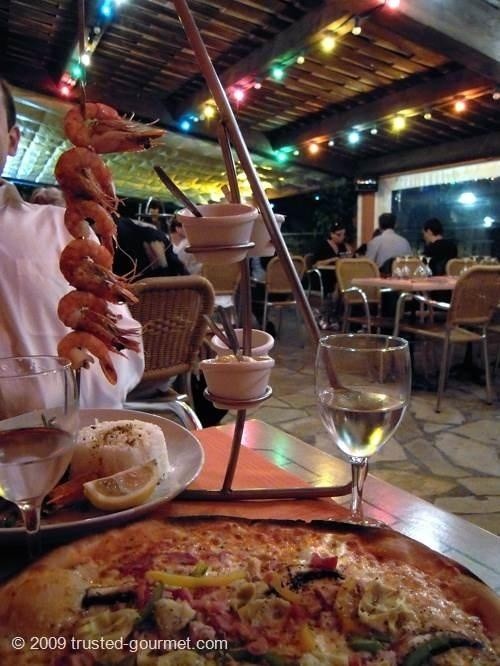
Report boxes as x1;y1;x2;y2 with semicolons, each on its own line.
0;519;500;666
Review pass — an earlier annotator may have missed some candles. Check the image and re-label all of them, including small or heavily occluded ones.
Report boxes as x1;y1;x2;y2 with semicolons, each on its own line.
351;274;463;334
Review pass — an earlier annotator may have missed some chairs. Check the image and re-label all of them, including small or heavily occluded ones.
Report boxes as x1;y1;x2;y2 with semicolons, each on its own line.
261;254;306;335
445;258;475;276
335;257;386;340
128;274;216;431
200;262;245;333
302;253;332;305
391;265;500;412
390;255;427;277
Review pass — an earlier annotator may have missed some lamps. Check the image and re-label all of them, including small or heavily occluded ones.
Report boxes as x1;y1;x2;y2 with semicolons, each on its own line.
233;89;243;101
308;142;320;155
71;65;83;78
272;67;283;79
292;148;299;157
347;130;360;145
327;139;335;147
60;84;70;98
454;97;470;117
296;55;306;66
491;91;499;101
385;0;402;13
180;120;191;132
390;115;406;132
253;82;262;91
370;127;377;136
351;24;363;36
320;36;337;54
423;110;434;119
204;105;214;119
80;51;92;66
100;3;114;19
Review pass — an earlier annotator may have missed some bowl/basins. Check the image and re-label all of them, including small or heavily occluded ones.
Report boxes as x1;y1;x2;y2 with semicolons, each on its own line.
247;213;286;258
199;356;276;411
174;203;259;265
207;327;274;356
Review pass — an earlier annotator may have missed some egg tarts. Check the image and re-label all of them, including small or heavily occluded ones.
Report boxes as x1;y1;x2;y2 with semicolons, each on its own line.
68;418;168;491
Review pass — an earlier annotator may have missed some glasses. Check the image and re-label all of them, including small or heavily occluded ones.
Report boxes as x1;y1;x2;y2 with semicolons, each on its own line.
335;232;349;237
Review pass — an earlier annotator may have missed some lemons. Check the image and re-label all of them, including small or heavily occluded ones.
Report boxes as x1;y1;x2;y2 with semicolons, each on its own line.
83;458;159;509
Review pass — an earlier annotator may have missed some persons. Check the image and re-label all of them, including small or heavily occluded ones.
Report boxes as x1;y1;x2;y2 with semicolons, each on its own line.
166;211;201;274
311;221;347;331
0;81;146;409
143;200;168;233
352;228;382;258
366;213;412;268
250;254;295;341
112;214;190;282
422;218;458;276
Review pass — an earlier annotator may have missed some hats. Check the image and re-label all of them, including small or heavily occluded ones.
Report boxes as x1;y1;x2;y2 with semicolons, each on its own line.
329;222;346;234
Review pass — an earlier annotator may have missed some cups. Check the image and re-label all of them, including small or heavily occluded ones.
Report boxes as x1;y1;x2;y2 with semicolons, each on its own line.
392;254;432;279
1;407;204;540
313;333;413;526
0;355;78;566
